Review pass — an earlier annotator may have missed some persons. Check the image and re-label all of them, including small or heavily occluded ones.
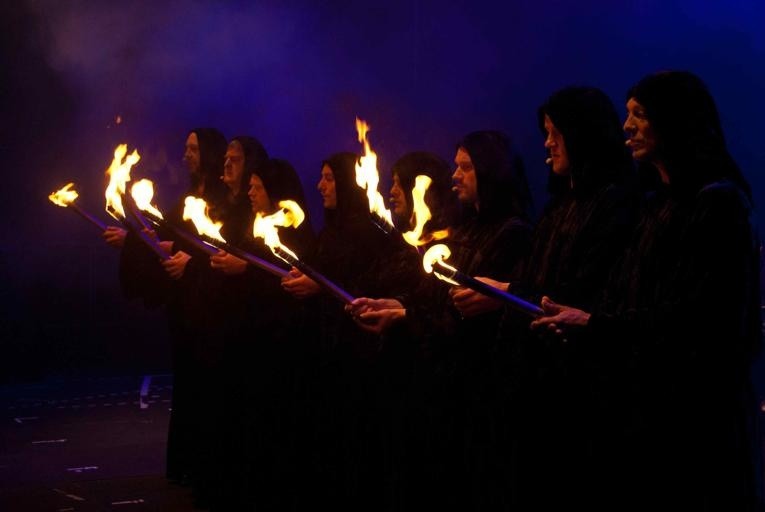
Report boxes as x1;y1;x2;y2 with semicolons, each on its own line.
112;135;257;512
166;162;332;512
349;128;526;512
101;124;219;509
283;150;450;512
206;153;375;512
528;69;763;511
444;85;638;505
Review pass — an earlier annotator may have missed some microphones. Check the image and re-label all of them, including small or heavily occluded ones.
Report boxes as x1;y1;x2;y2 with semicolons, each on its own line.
625;140;631;146
452;186;458;192
389;197;394;202
546;158;553;165
183;157;187;160
220;176;224;179
